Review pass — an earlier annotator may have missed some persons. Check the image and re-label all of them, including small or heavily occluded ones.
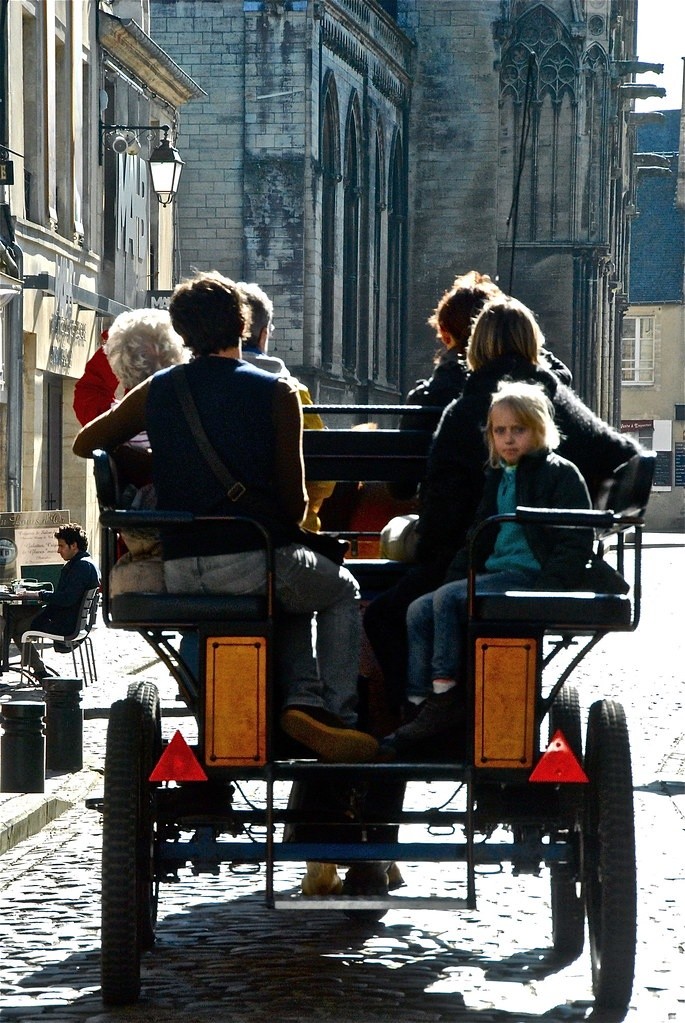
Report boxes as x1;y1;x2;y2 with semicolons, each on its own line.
394;373;594;747
0;522;100;686
71;280;353;567
72;268;396;767
363;270;641;732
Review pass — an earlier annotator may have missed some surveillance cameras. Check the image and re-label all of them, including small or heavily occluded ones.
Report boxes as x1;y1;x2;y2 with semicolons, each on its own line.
107;134;128;154
123;132;141;155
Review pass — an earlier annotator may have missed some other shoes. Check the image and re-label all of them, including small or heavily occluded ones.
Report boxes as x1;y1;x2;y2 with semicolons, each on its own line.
280;704;380;764
33;671;53;686
343;725;396;763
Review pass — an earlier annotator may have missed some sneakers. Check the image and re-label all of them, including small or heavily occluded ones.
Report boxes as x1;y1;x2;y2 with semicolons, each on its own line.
385;684;468;746
399;697;426;725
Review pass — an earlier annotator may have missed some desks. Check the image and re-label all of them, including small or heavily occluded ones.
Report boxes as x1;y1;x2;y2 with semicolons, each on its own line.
0;599;46;686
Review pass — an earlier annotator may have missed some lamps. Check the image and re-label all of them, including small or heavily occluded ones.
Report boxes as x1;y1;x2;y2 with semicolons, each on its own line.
98;87;185;208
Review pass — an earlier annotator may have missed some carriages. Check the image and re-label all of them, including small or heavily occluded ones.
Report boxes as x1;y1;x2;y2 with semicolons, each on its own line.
85;404;660;1023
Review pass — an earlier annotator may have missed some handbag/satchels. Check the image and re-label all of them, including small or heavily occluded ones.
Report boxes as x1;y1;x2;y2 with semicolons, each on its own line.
289;524;351;565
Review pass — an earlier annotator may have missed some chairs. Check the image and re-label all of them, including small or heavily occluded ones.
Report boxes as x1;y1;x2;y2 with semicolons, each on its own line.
20;586;100;688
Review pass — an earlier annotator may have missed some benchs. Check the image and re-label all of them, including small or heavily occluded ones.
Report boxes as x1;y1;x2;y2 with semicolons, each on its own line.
465;451;656;634
303;405;451;480
92;450;313;633
